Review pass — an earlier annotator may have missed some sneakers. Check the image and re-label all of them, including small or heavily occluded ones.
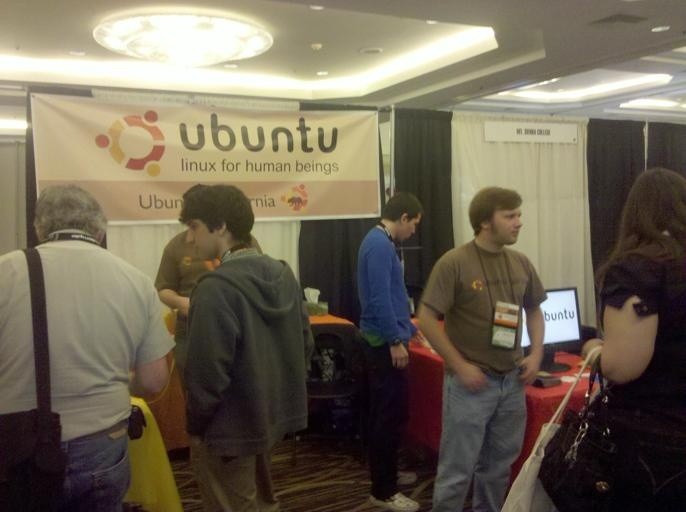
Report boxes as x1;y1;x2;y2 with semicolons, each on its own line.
393;471;417;485
368;488;421;512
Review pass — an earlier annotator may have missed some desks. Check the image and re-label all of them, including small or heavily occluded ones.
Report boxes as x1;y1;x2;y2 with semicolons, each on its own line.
409;318;596;492
120;397;185;512
135;304;357;455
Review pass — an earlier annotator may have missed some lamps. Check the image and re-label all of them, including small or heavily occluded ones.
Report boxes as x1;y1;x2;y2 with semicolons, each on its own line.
93;7;273;66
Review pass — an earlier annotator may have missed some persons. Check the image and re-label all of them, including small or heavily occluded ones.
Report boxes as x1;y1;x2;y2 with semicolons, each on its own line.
355;192;429;511
3;181;183;511
151;183;266;392
579;167;685;510
176;181;315;512
416;183;547;510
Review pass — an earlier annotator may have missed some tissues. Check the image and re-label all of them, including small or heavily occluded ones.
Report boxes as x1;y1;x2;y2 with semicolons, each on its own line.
302;287;329;317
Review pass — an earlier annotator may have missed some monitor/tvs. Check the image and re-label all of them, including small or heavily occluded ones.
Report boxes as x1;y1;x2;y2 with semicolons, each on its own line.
520;286;584;373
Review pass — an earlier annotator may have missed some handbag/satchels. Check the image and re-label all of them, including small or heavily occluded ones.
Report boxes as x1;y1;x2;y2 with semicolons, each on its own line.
538;354;625;508
0;406;67;512
498;345;603;512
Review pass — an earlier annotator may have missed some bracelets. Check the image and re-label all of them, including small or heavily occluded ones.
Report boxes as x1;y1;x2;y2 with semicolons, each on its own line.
390;338;402;346
